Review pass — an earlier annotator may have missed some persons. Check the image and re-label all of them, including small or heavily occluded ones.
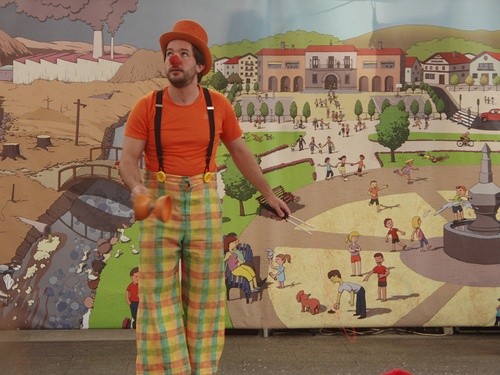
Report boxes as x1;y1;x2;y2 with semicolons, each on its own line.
119;18;292;375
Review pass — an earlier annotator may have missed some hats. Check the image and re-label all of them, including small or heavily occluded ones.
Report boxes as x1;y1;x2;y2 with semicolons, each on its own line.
159;20;212;76
